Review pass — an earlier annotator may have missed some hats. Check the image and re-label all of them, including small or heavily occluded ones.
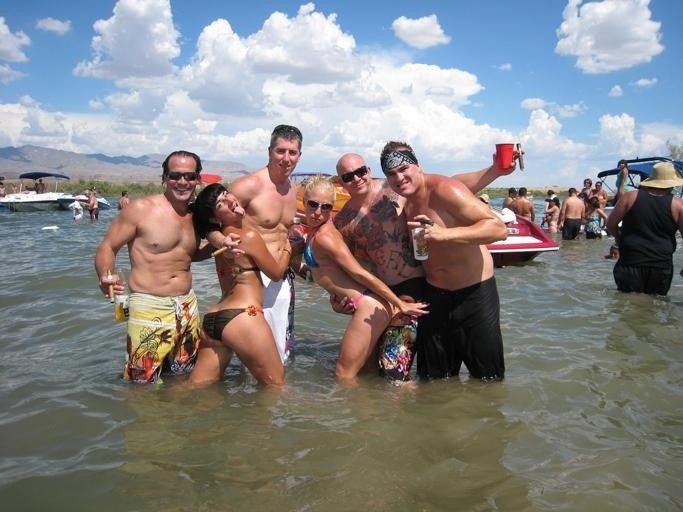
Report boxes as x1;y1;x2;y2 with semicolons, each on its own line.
479;194;491;203
638;162;683;188
545;194;559;202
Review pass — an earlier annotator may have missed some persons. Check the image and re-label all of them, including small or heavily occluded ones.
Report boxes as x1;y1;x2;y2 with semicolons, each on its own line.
330;149;524;390
94;150;217;385
380;141;509;383
206;124;312;366
302;176;431;388
0;179;129;222
169;183;306;400
606;161;683;295
479;159;629;259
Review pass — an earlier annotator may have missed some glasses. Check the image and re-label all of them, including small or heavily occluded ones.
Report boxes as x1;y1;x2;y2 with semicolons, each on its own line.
169;172;198;180
341;166;367;182
307;200;332;212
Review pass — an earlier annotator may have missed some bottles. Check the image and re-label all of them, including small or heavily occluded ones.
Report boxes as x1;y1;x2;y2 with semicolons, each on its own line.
289;218;303;242
114;267;129;322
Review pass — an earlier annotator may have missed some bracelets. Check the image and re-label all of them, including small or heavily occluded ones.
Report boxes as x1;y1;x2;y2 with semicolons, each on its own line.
283;248;291;256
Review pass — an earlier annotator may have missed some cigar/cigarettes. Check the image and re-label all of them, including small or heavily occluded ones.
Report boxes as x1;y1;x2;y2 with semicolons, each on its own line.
517;143;525;169
211;239;241;256
107;268;115;303
415;219;434;225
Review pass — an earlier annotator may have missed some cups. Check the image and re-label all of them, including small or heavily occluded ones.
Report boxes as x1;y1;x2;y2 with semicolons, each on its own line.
411;229;429;260
495;144;514;170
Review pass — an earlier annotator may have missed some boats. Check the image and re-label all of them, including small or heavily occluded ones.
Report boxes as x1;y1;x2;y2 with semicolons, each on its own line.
0;172;111;212
